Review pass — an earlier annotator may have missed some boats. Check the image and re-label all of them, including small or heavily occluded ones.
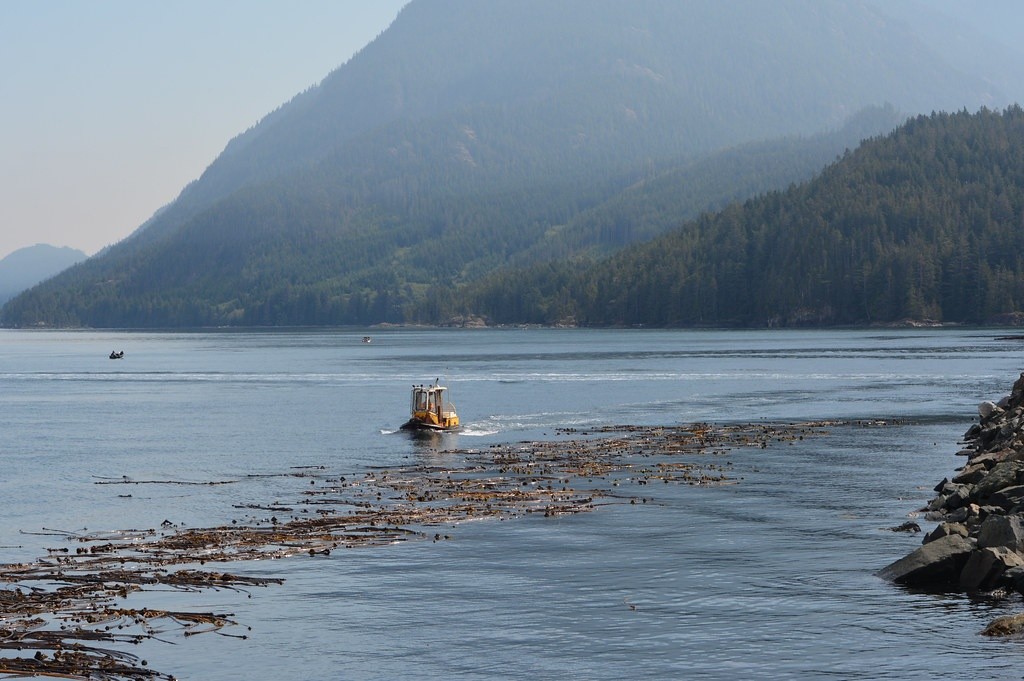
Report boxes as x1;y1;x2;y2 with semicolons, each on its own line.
362;336;372;343
399;376;459;431
110;350;124;359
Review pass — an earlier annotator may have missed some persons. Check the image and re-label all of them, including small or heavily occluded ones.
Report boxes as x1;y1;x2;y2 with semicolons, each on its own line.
421;396;434;412
